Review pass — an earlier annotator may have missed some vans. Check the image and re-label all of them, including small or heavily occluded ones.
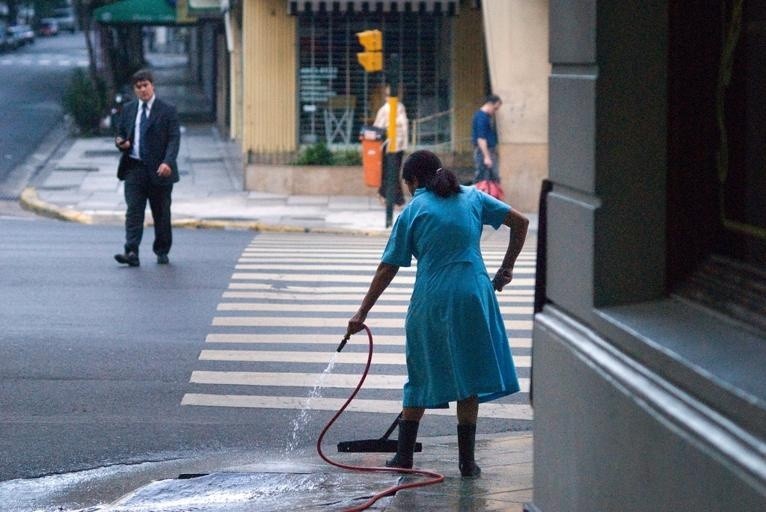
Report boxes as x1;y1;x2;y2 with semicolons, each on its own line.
52;8;79;33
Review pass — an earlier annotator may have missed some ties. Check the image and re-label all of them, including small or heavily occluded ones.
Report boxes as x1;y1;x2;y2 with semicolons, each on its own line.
139;103;148;159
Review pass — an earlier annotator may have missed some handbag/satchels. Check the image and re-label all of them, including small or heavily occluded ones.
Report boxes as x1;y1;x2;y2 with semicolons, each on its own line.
475;177;503;200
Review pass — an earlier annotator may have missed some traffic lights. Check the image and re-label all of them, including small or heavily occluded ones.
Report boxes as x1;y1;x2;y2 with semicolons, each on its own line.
353;27;383;74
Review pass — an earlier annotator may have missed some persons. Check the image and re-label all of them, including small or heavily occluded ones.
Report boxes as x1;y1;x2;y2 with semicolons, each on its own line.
114;69;180;267
372;84;409;208
347;150;530;476
471;95;501;183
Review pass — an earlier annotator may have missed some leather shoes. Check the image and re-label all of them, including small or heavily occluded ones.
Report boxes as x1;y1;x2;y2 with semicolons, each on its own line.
158;255;169;264
115;252;139;266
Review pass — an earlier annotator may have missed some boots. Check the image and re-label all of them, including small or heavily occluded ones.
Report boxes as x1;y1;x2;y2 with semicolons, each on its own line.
457;422;480;478
386;419;419;468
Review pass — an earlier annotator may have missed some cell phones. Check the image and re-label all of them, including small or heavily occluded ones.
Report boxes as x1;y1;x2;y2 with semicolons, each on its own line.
120;137;129;145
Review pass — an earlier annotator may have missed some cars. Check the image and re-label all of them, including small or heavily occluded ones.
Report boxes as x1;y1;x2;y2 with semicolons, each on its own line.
0;18;58;49
